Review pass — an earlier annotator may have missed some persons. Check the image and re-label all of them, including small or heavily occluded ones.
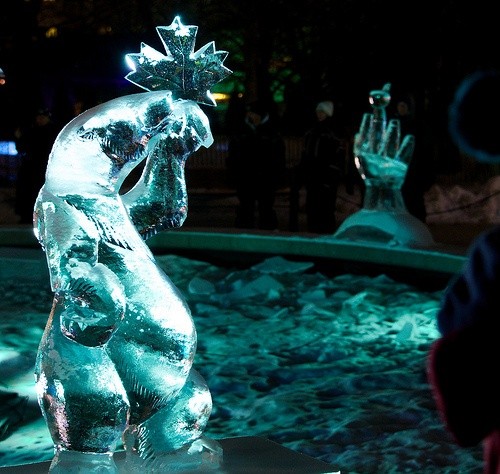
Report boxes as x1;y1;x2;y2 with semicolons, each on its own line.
1;55;499;271
412;174;500;474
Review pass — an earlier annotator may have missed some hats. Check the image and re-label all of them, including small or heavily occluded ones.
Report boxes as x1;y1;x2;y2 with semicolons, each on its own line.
316;100;336;115
